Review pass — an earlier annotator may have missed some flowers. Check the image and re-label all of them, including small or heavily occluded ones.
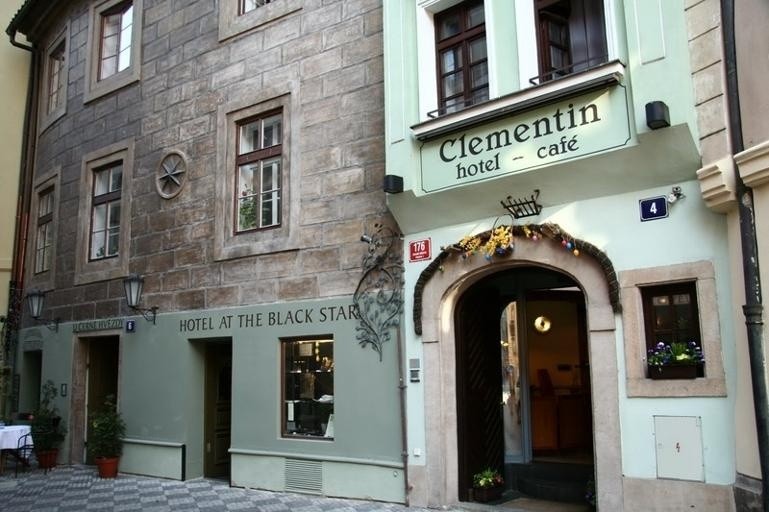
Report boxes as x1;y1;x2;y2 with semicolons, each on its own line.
643;334;707;372
458;226;515;261
474;466;504;487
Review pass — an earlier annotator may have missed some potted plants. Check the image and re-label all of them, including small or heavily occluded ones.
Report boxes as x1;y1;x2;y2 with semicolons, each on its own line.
32;377;69;471
84;393;128;480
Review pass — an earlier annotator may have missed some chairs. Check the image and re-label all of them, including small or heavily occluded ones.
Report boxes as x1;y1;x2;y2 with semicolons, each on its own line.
4;433;34;480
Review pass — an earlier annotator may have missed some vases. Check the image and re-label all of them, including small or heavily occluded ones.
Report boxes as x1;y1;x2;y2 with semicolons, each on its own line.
646;359;704;380
473;483;504;505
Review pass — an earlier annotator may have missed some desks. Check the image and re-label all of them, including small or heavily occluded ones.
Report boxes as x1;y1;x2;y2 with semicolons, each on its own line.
0;423;34;476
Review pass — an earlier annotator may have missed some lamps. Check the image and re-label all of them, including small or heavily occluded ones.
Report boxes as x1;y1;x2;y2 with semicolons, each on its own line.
122;270;160;326
23;285;59;335
